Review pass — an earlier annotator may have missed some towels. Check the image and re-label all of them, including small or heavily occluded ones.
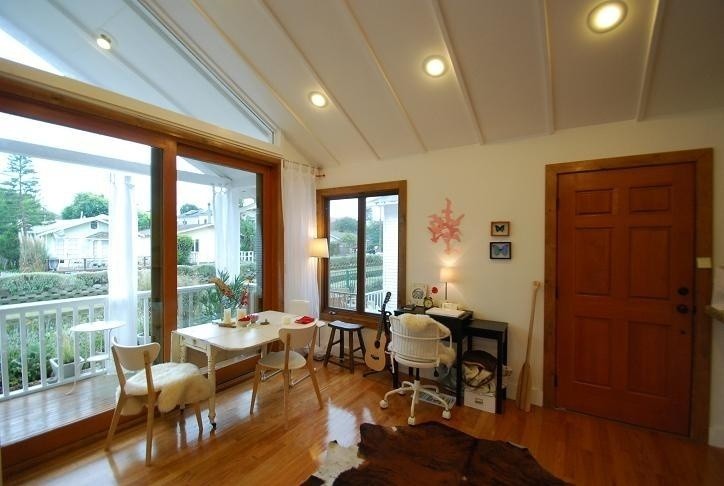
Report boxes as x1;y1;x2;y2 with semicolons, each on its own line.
295;315;315;324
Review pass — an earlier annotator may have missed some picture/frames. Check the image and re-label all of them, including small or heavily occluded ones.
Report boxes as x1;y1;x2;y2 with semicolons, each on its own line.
491;220;509;236
489;242;512;259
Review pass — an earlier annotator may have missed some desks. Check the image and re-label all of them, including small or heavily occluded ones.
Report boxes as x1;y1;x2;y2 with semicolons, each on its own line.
393;306;508;414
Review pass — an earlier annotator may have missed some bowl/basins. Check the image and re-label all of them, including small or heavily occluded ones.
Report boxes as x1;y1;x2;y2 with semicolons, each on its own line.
249;316;259;323
238;321;249;327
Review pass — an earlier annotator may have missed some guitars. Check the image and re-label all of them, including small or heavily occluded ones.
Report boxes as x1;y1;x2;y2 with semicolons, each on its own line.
364;292;392;372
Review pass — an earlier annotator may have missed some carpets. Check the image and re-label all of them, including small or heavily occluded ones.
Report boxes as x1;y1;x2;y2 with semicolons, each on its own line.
300;421;573;486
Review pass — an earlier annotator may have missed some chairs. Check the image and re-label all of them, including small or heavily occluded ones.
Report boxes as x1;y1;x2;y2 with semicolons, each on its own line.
103;336;213;467
250;318;324;430
380;314;457;426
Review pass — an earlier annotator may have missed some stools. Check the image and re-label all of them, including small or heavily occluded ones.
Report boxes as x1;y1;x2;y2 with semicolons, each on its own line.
65;321;126;396
325;319;366;374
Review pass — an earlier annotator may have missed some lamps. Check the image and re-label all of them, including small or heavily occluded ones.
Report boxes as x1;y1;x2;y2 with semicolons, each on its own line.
440;267;460;301
305;238;330;311
